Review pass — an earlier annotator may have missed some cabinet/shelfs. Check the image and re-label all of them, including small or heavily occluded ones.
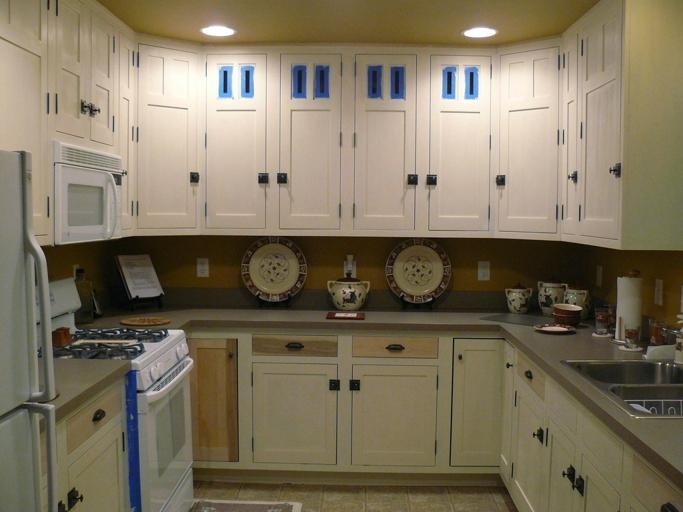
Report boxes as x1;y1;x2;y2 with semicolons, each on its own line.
492;34;558;240
248;323;441;474
37;353;133;511
204;46;347;240
42;0;135;151
555;1;683;252
443;334;502;469
135;31;204;237
187;336;241;464
620;437;682;512
351;42;491;238
515;357;628;511
500;340;516;501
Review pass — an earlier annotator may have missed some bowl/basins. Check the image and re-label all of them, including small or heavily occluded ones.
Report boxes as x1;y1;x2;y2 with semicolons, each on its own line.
550;303;582;328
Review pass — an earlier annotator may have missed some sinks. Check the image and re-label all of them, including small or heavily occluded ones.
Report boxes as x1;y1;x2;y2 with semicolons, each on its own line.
560;359;683;384
608;384;682;419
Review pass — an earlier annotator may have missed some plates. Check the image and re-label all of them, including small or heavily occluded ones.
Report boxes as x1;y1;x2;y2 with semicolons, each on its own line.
382;238;452;304
240;236;308;304
532;322;574;335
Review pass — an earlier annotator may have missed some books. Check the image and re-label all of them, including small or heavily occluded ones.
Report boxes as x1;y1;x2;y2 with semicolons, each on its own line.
117;253;165;300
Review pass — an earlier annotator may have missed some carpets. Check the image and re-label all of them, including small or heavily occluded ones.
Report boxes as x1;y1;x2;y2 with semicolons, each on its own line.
187;499;306;511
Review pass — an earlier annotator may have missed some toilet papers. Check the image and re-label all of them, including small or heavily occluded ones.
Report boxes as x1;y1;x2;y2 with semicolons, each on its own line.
614;276;644;342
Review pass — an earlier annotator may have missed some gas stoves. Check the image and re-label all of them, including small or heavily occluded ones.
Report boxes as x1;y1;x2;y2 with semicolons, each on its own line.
30;325;189;390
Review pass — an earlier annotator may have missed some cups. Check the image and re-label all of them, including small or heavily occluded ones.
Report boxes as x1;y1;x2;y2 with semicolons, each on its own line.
647;317;666;345
623;325;640;350
594;307;609;335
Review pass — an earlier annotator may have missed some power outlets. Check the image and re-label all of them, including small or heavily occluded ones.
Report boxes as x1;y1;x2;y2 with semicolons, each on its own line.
594;264;604;287
342;261;357;279
196;258;209;279
652;279;666;306
475;259;491;282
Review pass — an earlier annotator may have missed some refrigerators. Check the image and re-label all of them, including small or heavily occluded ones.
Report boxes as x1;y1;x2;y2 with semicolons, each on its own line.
2;150;61;510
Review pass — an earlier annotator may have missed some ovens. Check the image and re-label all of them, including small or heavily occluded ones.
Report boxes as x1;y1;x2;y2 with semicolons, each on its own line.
136;357;192;512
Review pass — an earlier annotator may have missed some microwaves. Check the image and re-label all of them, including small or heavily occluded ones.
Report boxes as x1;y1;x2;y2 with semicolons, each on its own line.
54;162;124;245
